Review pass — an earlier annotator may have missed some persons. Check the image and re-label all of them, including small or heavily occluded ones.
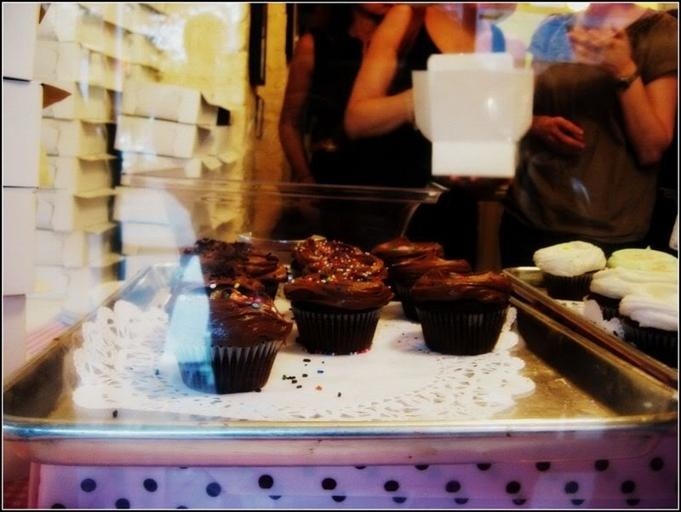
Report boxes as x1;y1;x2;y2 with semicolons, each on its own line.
496;3;678;272
274;2;396;244
343;3;484;267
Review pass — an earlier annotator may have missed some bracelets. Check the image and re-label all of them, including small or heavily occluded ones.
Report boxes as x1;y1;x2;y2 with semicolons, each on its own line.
404;87;413;127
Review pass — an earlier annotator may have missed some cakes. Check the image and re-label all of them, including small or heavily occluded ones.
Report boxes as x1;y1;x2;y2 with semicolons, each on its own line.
532;240;679;369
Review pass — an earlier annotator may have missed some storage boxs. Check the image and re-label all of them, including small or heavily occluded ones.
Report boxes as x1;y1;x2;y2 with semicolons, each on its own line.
0;1;249;345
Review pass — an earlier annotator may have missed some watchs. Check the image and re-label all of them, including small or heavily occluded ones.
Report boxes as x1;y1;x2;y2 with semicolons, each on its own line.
613;67;642;91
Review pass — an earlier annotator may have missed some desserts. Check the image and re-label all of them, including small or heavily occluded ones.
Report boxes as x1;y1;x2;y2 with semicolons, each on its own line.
372;239;511;355
183;236;293;395
283;234;395;356
